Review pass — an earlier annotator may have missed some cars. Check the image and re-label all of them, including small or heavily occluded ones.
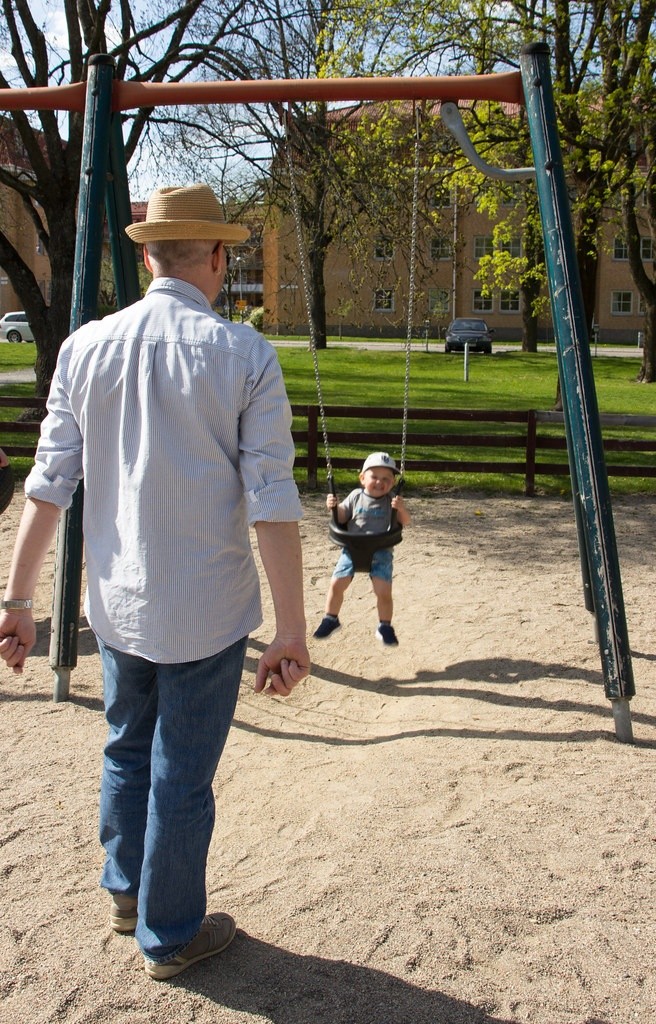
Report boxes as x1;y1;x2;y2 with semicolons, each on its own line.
442;317;495;355
0;311;35;344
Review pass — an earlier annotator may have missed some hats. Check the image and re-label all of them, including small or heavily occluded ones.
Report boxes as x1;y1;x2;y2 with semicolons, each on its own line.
361;452;400;475
124;183;251;244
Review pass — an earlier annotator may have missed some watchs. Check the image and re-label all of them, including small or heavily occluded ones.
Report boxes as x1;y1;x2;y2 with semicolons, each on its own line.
0;599;34;610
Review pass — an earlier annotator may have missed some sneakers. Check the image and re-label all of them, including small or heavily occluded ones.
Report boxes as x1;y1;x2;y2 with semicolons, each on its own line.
145;912;236;978
312;615;341;640
109;893;138;931
376;623;399;647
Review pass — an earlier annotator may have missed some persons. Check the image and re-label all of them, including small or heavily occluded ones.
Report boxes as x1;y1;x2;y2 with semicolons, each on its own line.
310;452;411;648
0;182;310;983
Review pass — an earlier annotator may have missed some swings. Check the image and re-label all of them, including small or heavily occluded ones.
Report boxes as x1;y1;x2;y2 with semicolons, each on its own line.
282;101;420;572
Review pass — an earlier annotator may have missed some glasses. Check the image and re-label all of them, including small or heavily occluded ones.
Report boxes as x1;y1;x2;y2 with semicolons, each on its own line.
211;241;232;267
